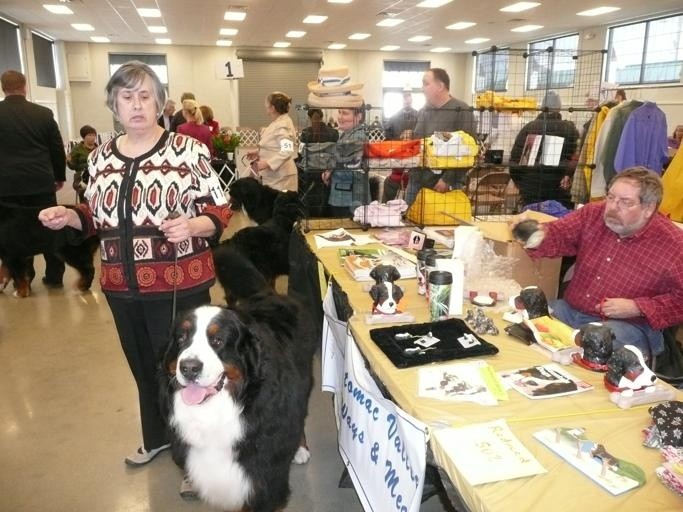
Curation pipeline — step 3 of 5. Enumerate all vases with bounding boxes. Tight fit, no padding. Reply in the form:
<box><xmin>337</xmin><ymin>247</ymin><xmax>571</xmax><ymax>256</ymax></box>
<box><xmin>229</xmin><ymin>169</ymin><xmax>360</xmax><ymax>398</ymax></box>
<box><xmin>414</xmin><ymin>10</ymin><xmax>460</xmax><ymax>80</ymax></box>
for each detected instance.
<box><xmin>216</xmin><ymin>148</ymin><xmax>234</xmax><ymax>159</ymax></box>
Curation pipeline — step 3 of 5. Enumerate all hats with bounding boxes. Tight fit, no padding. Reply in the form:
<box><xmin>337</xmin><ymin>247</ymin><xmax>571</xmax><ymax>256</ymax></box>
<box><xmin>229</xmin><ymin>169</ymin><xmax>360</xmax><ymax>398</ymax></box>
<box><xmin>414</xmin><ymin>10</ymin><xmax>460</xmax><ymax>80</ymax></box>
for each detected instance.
<box><xmin>541</xmin><ymin>91</ymin><xmax>561</xmax><ymax>108</ymax></box>
<box><xmin>307</xmin><ymin>63</ymin><xmax>363</xmax><ymax>108</ymax></box>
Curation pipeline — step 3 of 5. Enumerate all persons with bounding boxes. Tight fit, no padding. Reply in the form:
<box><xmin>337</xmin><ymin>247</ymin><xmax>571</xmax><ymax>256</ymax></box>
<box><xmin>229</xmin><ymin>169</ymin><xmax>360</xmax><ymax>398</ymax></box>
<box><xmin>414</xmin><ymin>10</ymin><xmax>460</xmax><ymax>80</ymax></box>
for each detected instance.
<box><xmin>247</xmin><ymin>92</ymin><xmax>298</xmax><ymax>192</ymax></box>
<box><xmin>0</xmin><ymin>70</ymin><xmax>67</xmax><ymax>289</ymax></box>
<box><xmin>299</xmin><ymin>109</ymin><xmax>339</xmax><ymax>143</ymax></box>
<box><xmin>511</xmin><ymin>165</ymin><xmax>683</xmax><ymax>359</ymax></box>
<box><xmin>37</xmin><ymin>60</ymin><xmax>234</xmax><ymax>503</ymax></box>
<box><xmin>508</xmin><ymin>91</ymin><xmax>579</xmax><ymax>210</ymax></box>
<box><xmin>405</xmin><ymin>70</ymin><xmax>478</xmax><ymax>206</ymax></box>
<box><xmin>387</xmin><ymin>94</ymin><xmax>418</xmax><ymax>140</ymax></box>
<box><xmin>668</xmin><ymin>125</ymin><xmax>683</xmax><ymax>141</ymax></box>
<box><xmin>322</xmin><ymin>94</ymin><xmax>372</xmax><ymax>218</ymax></box>
<box><xmin>67</xmin><ymin>125</ymin><xmax>99</xmax><ymax>203</ymax></box>
<box><xmin>157</xmin><ymin>92</ymin><xmax>221</xmax><ymax>159</ymax></box>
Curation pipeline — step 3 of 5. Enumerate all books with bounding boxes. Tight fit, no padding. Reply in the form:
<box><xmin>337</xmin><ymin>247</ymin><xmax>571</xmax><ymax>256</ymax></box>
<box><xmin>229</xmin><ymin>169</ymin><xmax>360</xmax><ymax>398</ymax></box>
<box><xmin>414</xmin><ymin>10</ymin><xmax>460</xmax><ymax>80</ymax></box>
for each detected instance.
<box><xmin>519</xmin><ymin>135</ymin><xmax>543</xmax><ymax>165</ymax></box>
<box><xmin>338</xmin><ymin>248</ymin><xmax>419</xmax><ymax>282</ymax></box>
<box><xmin>503</xmin><ymin>363</ymin><xmax>594</xmax><ymax>399</ymax></box>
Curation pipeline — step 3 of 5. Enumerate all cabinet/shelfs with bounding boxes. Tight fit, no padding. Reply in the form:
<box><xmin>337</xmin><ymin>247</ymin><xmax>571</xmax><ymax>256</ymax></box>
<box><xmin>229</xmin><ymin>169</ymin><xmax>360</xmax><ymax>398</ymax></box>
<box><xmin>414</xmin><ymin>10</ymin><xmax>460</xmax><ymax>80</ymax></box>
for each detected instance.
<box><xmin>296</xmin><ymin>47</ymin><xmax>607</xmax><ymax>234</ymax></box>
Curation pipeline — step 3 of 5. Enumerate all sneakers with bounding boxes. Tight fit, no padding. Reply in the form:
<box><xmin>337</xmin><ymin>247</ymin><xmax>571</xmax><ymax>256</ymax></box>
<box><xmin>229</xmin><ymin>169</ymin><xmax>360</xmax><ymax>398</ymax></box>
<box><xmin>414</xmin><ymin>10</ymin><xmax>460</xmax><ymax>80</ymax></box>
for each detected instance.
<box><xmin>125</xmin><ymin>441</ymin><xmax>171</xmax><ymax>467</ymax></box>
<box><xmin>180</xmin><ymin>475</ymin><xmax>202</xmax><ymax>500</ymax></box>
<box><xmin>0</xmin><ymin>263</ymin><xmax>94</xmax><ymax>298</ymax></box>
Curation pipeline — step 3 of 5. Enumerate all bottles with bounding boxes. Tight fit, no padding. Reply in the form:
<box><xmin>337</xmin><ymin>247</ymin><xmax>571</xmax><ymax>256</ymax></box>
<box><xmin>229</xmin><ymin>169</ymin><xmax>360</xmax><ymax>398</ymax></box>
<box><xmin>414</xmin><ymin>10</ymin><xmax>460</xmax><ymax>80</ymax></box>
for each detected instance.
<box><xmin>493</xmin><ymin>153</ymin><xmax>502</xmax><ymax>165</ymax></box>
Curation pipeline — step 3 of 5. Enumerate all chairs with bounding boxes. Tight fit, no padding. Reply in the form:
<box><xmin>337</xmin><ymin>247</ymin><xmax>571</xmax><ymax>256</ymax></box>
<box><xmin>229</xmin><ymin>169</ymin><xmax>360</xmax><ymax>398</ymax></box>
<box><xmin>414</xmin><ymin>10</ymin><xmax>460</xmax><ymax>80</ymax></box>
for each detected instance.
<box><xmin>469</xmin><ymin>172</ymin><xmax>511</xmax><ymax>217</ymax></box>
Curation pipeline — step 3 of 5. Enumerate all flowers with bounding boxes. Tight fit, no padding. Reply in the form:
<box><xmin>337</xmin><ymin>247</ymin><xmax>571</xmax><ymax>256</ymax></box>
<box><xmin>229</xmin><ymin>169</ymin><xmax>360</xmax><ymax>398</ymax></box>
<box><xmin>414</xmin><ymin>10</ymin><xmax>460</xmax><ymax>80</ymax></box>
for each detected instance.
<box><xmin>211</xmin><ymin>129</ymin><xmax>241</xmax><ymax>152</ymax></box>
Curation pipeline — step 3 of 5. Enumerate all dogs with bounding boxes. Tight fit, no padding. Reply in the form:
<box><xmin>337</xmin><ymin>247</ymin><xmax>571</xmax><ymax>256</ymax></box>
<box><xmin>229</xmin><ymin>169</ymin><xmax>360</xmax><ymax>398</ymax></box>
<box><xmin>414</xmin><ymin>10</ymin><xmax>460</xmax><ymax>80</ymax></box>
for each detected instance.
<box><xmin>370</xmin><ymin>262</ymin><xmax>402</xmax><ymax>283</ymax></box>
<box><xmin>227</xmin><ymin>175</ymin><xmax>283</xmax><ymax>226</ymax></box>
<box><xmin>574</xmin><ymin>321</ymin><xmax>616</xmax><ymax>371</ymax></box>
<box><xmin>76</xmin><ymin>170</ymin><xmax>89</xmax><ymax>191</ymax></box>
<box><xmin>514</xmin><ymin>286</ymin><xmax>552</xmax><ymax>323</ymax></box>
<box><xmin>153</xmin><ymin>245</ymin><xmax>317</xmax><ymax>511</ymax></box>
<box><xmin>0</xmin><ymin>205</ymin><xmax>100</xmax><ymax>298</ymax></box>
<box><xmin>605</xmin><ymin>344</ymin><xmax>657</xmax><ymax>390</ymax></box>
<box><xmin>367</xmin><ymin>281</ymin><xmax>405</xmax><ymax>316</ymax></box>
<box><xmin>213</xmin><ymin>189</ymin><xmax>313</xmax><ymax>308</ymax></box>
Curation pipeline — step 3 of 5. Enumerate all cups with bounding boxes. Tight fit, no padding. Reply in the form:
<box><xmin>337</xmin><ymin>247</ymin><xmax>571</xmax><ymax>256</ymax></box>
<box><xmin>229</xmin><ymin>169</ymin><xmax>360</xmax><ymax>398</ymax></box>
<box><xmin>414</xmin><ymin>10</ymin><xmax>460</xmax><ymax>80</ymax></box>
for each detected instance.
<box><xmin>416</xmin><ymin>249</ymin><xmax>452</xmax><ymax>323</ymax></box>
<box><xmin>227</xmin><ymin>152</ymin><xmax>233</xmax><ymax>160</ymax></box>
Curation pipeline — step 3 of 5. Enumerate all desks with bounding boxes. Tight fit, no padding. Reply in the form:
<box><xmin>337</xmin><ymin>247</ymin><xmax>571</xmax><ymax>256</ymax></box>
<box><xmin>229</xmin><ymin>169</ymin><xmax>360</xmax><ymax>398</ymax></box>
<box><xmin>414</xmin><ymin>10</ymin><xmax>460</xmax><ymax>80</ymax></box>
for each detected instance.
<box><xmin>292</xmin><ymin>211</ymin><xmax>683</xmax><ymax>512</ymax></box>
<box><xmin>211</xmin><ymin>159</ymin><xmax>236</xmax><ymax>191</ymax></box>
